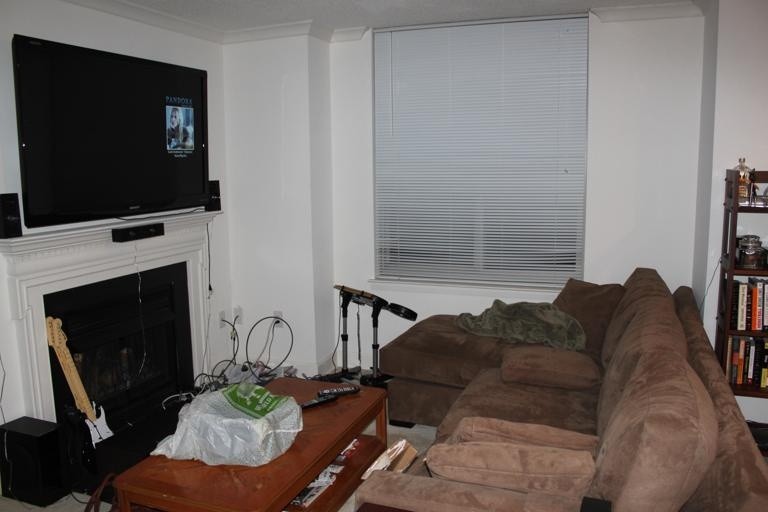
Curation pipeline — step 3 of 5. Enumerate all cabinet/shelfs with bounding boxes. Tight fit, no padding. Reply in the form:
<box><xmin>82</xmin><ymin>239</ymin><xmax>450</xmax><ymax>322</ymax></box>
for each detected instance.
<box><xmin>717</xmin><ymin>168</ymin><xmax>768</xmax><ymax>457</ymax></box>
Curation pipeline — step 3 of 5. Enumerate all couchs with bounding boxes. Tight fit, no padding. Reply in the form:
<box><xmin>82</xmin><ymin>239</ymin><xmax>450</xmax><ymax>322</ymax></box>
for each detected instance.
<box><xmin>354</xmin><ymin>268</ymin><xmax>768</xmax><ymax>512</ymax></box>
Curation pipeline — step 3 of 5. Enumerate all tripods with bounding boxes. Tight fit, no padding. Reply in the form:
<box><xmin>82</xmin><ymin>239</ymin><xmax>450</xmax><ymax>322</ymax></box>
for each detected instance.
<box><xmin>325</xmin><ymin>296</ymin><xmax>360</xmax><ymax>381</ymax></box>
<box><xmin>360</xmin><ymin>309</ymin><xmax>393</xmax><ymax>391</ymax></box>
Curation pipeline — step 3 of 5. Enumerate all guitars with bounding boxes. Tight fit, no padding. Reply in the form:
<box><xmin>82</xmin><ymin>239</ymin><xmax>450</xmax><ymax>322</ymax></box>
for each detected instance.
<box><xmin>46</xmin><ymin>317</ymin><xmax>115</xmax><ymax>471</ymax></box>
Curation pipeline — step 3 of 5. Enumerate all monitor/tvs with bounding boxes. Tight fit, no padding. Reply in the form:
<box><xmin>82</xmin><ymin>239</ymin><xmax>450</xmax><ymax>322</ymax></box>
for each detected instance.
<box><xmin>11</xmin><ymin>34</ymin><xmax>211</xmax><ymax>230</ymax></box>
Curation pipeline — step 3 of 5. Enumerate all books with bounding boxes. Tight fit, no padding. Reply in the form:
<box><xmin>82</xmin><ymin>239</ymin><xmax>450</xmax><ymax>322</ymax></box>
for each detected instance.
<box><xmin>288</xmin><ymin>463</ymin><xmax>345</xmax><ymax>508</ymax></box>
<box><xmin>730</xmin><ymin>337</ymin><xmax>768</xmax><ymax>387</ymax></box>
<box><xmin>731</xmin><ymin>277</ymin><xmax>768</xmax><ymax>331</ymax></box>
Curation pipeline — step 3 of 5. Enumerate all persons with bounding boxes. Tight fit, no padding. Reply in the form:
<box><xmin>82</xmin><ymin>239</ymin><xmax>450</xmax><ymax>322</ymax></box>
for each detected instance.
<box><xmin>165</xmin><ymin>106</ymin><xmax>190</xmax><ymax>149</ymax></box>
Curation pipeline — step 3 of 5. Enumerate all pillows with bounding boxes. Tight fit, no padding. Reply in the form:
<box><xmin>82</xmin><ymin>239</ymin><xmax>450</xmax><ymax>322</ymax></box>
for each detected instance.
<box><xmin>595</xmin><ymin>350</ymin><xmax>718</xmax><ymax>512</ymax></box>
<box><xmin>502</xmin><ymin>342</ymin><xmax>606</xmax><ymax>391</ymax></box>
<box><xmin>446</xmin><ymin>417</ymin><xmax>601</xmax><ymax>460</ymax></box>
<box><xmin>590</xmin><ymin>303</ymin><xmax>687</xmax><ymax>439</ymax></box>
<box><xmin>426</xmin><ymin>443</ymin><xmax>595</xmax><ymax>500</ymax></box>
<box><xmin>600</xmin><ymin>268</ymin><xmax>677</xmax><ymax>373</ymax></box>
<box><xmin>547</xmin><ymin>276</ymin><xmax>625</xmax><ymax>352</ymax></box>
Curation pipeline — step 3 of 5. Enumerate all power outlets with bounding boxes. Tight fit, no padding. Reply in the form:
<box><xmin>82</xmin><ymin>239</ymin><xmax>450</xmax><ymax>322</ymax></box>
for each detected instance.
<box><xmin>272</xmin><ymin>310</ymin><xmax>282</xmax><ymax>328</ymax></box>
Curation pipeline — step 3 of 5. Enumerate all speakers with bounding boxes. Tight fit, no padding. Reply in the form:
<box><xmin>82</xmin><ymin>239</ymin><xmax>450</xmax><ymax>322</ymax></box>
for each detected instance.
<box><xmin>0</xmin><ymin>415</ymin><xmax>73</xmax><ymax>508</ymax></box>
<box><xmin>203</xmin><ymin>179</ymin><xmax>222</xmax><ymax>212</ymax></box>
<box><xmin>0</xmin><ymin>191</ymin><xmax>23</xmax><ymax>239</ymax></box>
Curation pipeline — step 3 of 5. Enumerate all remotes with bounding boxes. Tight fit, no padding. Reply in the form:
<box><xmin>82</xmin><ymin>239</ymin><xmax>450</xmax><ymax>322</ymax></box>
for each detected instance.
<box><xmin>318</xmin><ymin>385</ymin><xmax>360</xmax><ymax>396</ymax></box>
<box><xmin>299</xmin><ymin>393</ymin><xmax>336</xmax><ymax>409</ymax></box>
